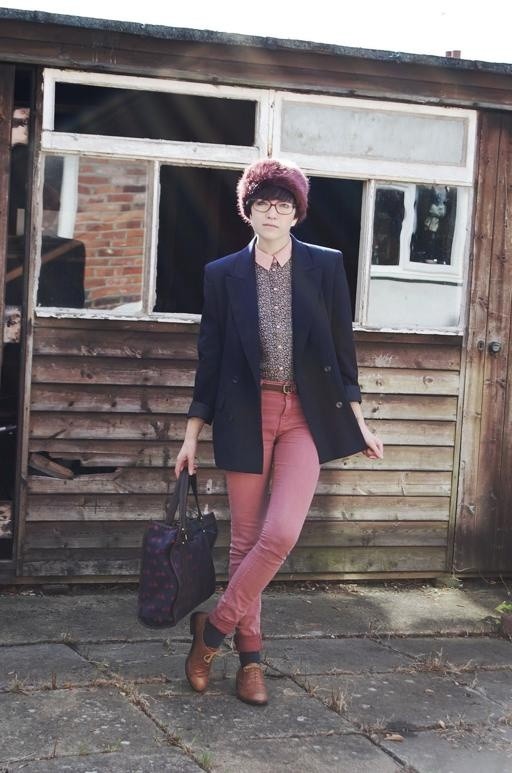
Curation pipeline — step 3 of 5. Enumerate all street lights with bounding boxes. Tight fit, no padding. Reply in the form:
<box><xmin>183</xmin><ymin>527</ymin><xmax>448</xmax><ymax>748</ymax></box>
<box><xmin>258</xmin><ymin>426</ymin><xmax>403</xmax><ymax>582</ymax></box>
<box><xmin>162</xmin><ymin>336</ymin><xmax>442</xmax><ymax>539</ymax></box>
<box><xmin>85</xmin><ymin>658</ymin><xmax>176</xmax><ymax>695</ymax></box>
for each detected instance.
<box><xmin>134</xmin><ymin>466</ymin><xmax>218</xmax><ymax>631</ymax></box>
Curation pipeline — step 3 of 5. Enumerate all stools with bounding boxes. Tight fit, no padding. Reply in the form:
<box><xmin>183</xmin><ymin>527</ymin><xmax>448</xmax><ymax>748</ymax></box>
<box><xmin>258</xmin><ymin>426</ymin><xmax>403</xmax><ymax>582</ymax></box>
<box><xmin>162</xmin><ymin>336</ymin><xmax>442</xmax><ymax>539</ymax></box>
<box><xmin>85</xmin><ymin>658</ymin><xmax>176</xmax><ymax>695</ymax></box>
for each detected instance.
<box><xmin>262</xmin><ymin>383</ymin><xmax>298</xmax><ymax>394</ymax></box>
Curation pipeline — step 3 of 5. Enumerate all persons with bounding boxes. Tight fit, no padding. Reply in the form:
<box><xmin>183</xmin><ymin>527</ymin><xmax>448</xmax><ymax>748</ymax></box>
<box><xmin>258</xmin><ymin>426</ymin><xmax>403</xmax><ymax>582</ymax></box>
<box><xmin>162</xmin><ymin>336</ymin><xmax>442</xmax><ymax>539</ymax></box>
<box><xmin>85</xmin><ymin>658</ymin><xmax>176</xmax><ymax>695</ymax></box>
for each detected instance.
<box><xmin>172</xmin><ymin>160</ymin><xmax>384</xmax><ymax>705</ymax></box>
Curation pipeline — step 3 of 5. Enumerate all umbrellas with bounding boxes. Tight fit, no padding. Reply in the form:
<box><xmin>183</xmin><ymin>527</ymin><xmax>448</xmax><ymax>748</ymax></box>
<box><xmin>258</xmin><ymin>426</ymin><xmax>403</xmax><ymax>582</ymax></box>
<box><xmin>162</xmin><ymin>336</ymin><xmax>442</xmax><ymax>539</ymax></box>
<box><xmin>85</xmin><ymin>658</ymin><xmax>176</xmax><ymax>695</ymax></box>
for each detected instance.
<box><xmin>233</xmin><ymin>663</ymin><xmax>268</xmax><ymax>708</ymax></box>
<box><xmin>185</xmin><ymin>609</ymin><xmax>220</xmax><ymax>695</ymax></box>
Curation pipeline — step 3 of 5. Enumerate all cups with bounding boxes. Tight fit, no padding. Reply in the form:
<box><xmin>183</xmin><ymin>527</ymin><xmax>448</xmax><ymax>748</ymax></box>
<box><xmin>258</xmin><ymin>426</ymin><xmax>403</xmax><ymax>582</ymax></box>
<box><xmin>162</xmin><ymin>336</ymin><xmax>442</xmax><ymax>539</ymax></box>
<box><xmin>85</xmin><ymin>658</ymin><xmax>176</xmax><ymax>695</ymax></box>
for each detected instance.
<box><xmin>250</xmin><ymin>196</ymin><xmax>297</xmax><ymax>215</ymax></box>
